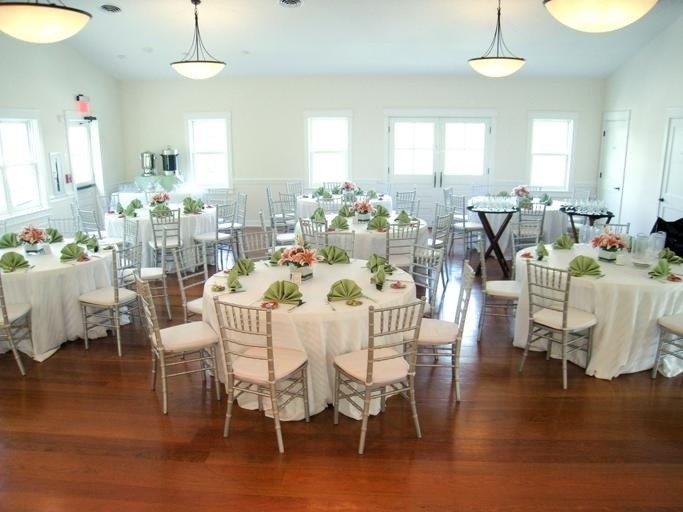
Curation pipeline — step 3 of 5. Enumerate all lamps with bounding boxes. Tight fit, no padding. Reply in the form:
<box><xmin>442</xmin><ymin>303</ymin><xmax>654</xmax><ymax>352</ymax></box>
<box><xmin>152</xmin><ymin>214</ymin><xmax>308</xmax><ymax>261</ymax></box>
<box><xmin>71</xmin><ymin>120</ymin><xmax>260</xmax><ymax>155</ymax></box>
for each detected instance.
<box><xmin>0</xmin><ymin>0</ymin><xmax>93</xmax><ymax>43</ymax></box>
<box><xmin>78</xmin><ymin>94</ymin><xmax>89</xmax><ymax>113</ymax></box>
<box><xmin>543</xmin><ymin>0</ymin><xmax>659</xmax><ymax>33</ymax></box>
<box><xmin>170</xmin><ymin>0</ymin><xmax>227</xmax><ymax>80</ymax></box>
<box><xmin>468</xmin><ymin>0</ymin><xmax>526</xmax><ymax>78</ymax></box>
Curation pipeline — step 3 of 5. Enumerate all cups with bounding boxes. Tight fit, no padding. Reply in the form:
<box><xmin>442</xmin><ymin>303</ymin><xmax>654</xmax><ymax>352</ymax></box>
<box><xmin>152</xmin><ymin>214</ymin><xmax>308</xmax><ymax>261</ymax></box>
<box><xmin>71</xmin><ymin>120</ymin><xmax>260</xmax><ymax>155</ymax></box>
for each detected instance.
<box><xmin>561</xmin><ymin>199</ymin><xmax>608</xmax><ymax>217</ymax></box>
<box><xmin>472</xmin><ymin>197</ymin><xmax>519</xmax><ymax>211</ymax></box>
